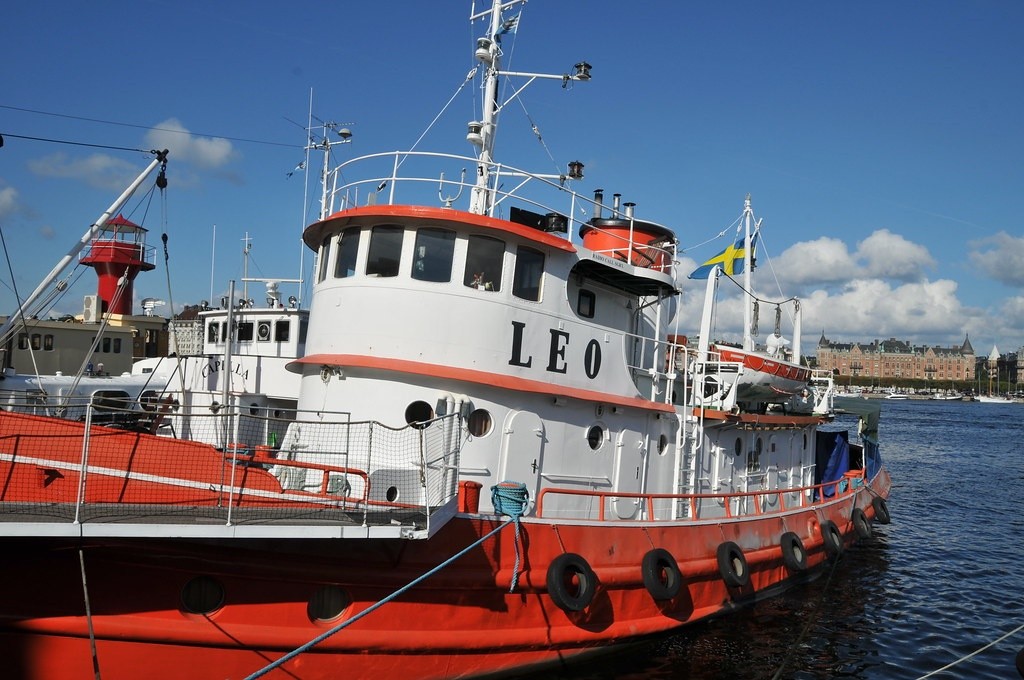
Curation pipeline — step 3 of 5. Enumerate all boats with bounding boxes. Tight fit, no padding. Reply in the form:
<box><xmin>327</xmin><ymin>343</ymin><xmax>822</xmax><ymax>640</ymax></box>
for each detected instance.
<box><xmin>0</xmin><ymin>0</ymin><xmax>1024</xmax><ymax>679</ymax></box>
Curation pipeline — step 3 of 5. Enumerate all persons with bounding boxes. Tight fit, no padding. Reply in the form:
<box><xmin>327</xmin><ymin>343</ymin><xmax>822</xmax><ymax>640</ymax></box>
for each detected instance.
<box><xmin>86</xmin><ymin>362</ymin><xmax>94</xmax><ymax>376</ymax></box>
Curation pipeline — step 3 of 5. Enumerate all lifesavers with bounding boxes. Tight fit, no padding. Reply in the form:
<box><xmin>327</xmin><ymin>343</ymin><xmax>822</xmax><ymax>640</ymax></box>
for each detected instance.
<box><xmin>716</xmin><ymin>541</ymin><xmax>750</xmax><ymax>587</ymax></box>
<box><xmin>820</xmin><ymin>520</ymin><xmax>844</xmax><ymax>557</ymax></box>
<box><xmin>872</xmin><ymin>496</ymin><xmax>890</xmax><ymax>525</ymax></box>
<box><xmin>780</xmin><ymin>531</ymin><xmax>808</xmax><ymax>571</ymax></box>
<box><xmin>547</xmin><ymin>553</ymin><xmax>598</xmax><ymax>614</ymax></box>
<box><xmin>852</xmin><ymin>508</ymin><xmax>872</xmax><ymax>539</ymax></box>
<box><xmin>641</xmin><ymin>548</ymin><xmax>682</xmax><ymax>603</ymax></box>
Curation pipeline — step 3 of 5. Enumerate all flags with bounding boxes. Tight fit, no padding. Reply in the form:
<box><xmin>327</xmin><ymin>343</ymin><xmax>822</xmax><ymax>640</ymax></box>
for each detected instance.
<box><xmin>687</xmin><ymin>232</ymin><xmax>757</xmax><ymax>279</ymax></box>
